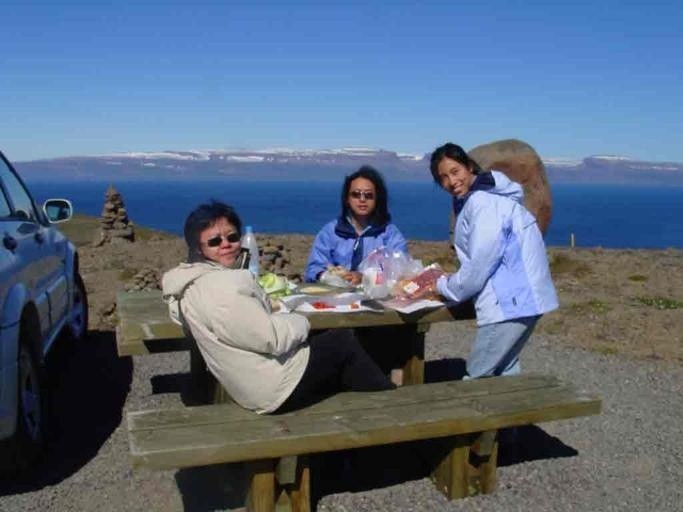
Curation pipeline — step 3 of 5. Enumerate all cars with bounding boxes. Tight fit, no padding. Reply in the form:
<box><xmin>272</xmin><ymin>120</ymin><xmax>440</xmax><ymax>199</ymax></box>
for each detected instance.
<box><xmin>0</xmin><ymin>151</ymin><xmax>88</xmax><ymax>444</ymax></box>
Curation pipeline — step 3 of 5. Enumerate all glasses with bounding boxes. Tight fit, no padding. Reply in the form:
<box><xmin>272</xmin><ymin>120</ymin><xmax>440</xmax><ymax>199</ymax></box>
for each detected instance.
<box><xmin>208</xmin><ymin>233</ymin><xmax>240</xmax><ymax>246</ymax></box>
<box><xmin>350</xmin><ymin>191</ymin><xmax>373</xmax><ymax>199</ymax></box>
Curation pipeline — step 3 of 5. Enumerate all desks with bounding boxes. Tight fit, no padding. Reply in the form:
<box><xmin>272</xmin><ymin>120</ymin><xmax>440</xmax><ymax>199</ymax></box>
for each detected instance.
<box><xmin>115</xmin><ymin>291</ymin><xmax>477</xmax><ymax>405</ymax></box>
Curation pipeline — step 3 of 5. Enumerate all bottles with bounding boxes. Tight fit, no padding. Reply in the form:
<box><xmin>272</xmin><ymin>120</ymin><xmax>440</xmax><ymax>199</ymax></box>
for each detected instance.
<box><xmin>240</xmin><ymin>225</ymin><xmax>260</xmax><ymax>281</ymax></box>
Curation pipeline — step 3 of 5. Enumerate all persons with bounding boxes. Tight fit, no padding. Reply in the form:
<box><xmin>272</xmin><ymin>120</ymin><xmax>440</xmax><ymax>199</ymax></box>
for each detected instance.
<box><xmin>304</xmin><ymin>165</ymin><xmax>410</xmax><ymax>387</ymax></box>
<box><xmin>161</xmin><ymin>199</ymin><xmax>414</xmax><ymax>488</ymax></box>
<box><xmin>429</xmin><ymin>143</ymin><xmax>560</xmax><ymax>443</ymax></box>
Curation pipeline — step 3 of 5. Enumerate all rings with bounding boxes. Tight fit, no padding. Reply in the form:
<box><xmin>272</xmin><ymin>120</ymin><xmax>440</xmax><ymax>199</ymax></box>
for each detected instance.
<box><xmin>429</xmin><ymin>288</ymin><xmax>433</xmax><ymax>292</ymax></box>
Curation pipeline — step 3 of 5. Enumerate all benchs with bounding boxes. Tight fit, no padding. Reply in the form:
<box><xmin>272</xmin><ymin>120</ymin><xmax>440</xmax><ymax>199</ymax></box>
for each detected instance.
<box><xmin>116</xmin><ymin>327</ymin><xmax>207</xmax><ymax>404</ymax></box>
<box><xmin>126</xmin><ymin>372</ymin><xmax>602</xmax><ymax>512</ymax></box>
